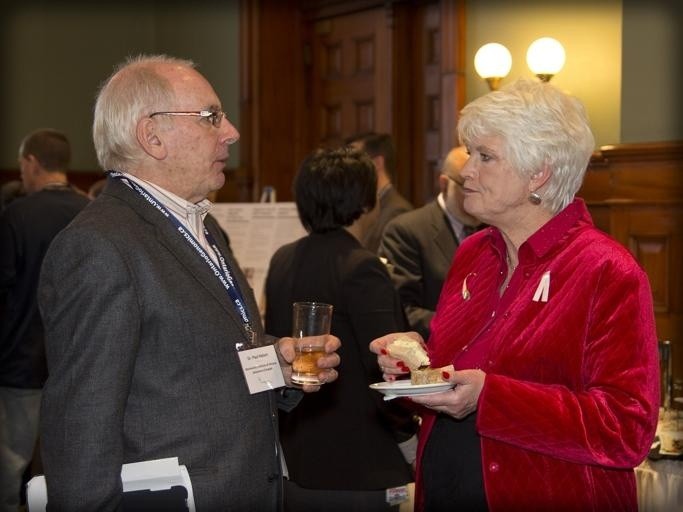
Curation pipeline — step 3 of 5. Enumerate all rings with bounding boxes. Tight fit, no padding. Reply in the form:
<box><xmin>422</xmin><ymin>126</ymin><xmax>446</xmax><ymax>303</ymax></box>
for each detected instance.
<box><xmin>379</xmin><ymin>365</ymin><xmax>384</xmax><ymax>371</ymax></box>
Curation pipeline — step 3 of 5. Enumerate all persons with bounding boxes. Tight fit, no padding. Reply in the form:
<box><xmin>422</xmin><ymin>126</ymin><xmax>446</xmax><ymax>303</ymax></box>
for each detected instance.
<box><xmin>0</xmin><ymin>129</ymin><xmax>96</xmax><ymax>511</ymax></box>
<box><xmin>366</xmin><ymin>77</ymin><xmax>663</xmax><ymax>511</ymax></box>
<box><xmin>344</xmin><ymin>132</ymin><xmax>419</xmax><ymax>260</ymax></box>
<box><xmin>84</xmin><ymin>177</ymin><xmax>106</xmax><ymax>200</ymax></box>
<box><xmin>378</xmin><ymin>145</ymin><xmax>492</xmax><ymax>346</ymax></box>
<box><xmin>261</xmin><ymin>144</ymin><xmax>419</xmax><ymax>512</ymax></box>
<box><xmin>0</xmin><ymin>178</ymin><xmax>26</xmax><ymax>208</ymax></box>
<box><xmin>38</xmin><ymin>54</ymin><xmax>344</xmax><ymax>510</ymax></box>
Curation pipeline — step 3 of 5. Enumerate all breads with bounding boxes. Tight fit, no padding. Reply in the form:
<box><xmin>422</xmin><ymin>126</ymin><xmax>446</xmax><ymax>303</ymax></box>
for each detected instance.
<box><xmin>387</xmin><ymin>337</ymin><xmax>455</xmax><ymax>385</ymax></box>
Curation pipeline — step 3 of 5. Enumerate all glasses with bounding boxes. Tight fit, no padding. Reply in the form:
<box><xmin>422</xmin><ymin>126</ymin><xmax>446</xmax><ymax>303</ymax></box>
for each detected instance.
<box><xmin>149</xmin><ymin>109</ymin><xmax>223</xmax><ymax>129</ymax></box>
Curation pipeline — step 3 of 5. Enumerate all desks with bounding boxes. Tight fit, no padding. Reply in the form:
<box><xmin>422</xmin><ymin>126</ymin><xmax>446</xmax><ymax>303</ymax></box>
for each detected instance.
<box><xmin>633</xmin><ymin>430</ymin><xmax>683</xmax><ymax>512</ymax></box>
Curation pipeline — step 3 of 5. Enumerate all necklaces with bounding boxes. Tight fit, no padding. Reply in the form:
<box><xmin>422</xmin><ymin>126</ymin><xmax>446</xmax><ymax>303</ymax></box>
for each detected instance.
<box><xmin>502</xmin><ymin>247</ymin><xmax>518</xmax><ymax>273</ymax></box>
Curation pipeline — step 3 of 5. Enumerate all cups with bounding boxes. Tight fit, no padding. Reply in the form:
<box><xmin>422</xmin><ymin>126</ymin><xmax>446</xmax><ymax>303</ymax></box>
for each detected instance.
<box><xmin>288</xmin><ymin>300</ymin><xmax>331</xmax><ymax>386</ymax></box>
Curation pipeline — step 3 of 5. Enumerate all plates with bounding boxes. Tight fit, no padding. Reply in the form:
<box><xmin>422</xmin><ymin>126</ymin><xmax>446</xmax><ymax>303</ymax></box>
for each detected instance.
<box><xmin>368</xmin><ymin>379</ymin><xmax>456</xmax><ymax>396</ymax></box>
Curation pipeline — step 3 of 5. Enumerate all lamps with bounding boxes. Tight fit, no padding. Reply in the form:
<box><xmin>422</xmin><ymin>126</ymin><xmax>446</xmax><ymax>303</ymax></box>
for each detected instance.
<box><xmin>526</xmin><ymin>39</ymin><xmax>567</xmax><ymax>83</ymax></box>
<box><xmin>474</xmin><ymin>43</ymin><xmax>512</xmax><ymax>92</ymax></box>
